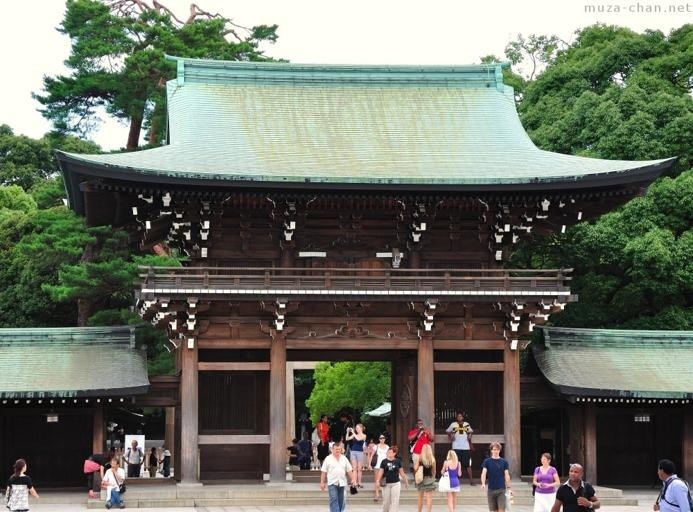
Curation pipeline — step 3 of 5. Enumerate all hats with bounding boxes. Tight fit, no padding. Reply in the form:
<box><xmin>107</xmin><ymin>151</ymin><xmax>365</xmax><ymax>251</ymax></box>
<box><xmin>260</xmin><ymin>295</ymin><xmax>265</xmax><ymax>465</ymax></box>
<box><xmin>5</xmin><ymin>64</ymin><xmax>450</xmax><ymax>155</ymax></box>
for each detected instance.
<box><xmin>163</xmin><ymin>450</ymin><xmax>171</xmax><ymax>457</ymax></box>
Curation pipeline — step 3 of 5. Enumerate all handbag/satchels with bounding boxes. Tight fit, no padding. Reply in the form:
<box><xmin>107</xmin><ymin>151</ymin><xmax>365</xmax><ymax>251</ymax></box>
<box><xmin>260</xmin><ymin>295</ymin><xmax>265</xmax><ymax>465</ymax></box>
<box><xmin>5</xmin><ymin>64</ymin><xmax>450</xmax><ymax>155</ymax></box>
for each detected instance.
<box><xmin>415</xmin><ymin>466</ymin><xmax>424</xmax><ymax>486</ymax></box>
<box><xmin>370</xmin><ymin>454</ymin><xmax>377</xmax><ymax>467</ymax></box>
<box><xmin>119</xmin><ymin>484</ymin><xmax>126</xmax><ymax>492</ymax></box>
<box><xmin>438</xmin><ymin>472</ymin><xmax>450</xmax><ymax>492</ymax></box>
<box><xmin>381</xmin><ymin>476</ymin><xmax>386</xmax><ymax>487</ymax></box>
<box><xmin>408</xmin><ymin>442</ymin><xmax>416</xmax><ymax>453</ymax></box>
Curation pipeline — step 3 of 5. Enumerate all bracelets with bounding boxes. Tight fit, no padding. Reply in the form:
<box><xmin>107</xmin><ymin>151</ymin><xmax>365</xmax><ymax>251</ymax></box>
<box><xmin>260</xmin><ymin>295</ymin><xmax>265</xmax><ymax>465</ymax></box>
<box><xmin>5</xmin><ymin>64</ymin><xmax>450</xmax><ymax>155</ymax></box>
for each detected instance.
<box><xmin>589</xmin><ymin>502</ymin><xmax>592</xmax><ymax>508</ymax></box>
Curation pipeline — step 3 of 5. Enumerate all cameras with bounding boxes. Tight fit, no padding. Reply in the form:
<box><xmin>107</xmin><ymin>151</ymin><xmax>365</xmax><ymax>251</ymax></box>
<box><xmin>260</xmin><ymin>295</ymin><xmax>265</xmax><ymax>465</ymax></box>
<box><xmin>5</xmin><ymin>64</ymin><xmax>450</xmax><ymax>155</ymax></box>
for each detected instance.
<box><xmin>348</xmin><ymin>428</ymin><xmax>352</xmax><ymax>431</ymax></box>
<box><xmin>420</xmin><ymin>427</ymin><xmax>424</xmax><ymax>430</ymax></box>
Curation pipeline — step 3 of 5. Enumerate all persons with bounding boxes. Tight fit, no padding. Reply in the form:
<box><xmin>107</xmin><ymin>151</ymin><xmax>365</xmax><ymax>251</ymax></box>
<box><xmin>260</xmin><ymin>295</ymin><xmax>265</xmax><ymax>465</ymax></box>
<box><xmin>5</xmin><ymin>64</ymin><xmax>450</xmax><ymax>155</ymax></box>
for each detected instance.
<box><xmin>159</xmin><ymin>444</ymin><xmax>171</xmax><ymax>477</ymax></box>
<box><xmin>287</xmin><ymin>413</ymin><xmax>353</xmax><ymax>470</ymax></box>
<box><xmin>441</xmin><ymin>450</ymin><xmax>462</xmax><ymax>512</ymax></box>
<box><xmin>414</xmin><ymin>444</ymin><xmax>436</xmax><ymax>512</ymax></box>
<box><xmin>445</xmin><ymin>411</ymin><xmax>476</xmax><ymax>486</ymax></box>
<box><xmin>533</xmin><ymin>453</ymin><xmax>561</xmax><ymax>512</ymax></box>
<box><xmin>368</xmin><ymin>433</ymin><xmax>390</xmax><ymax>502</ymax></box>
<box><xmin>320</xmin><ymin>443</ymin><xmax>356</xmax><ymax>512</ymax></box>
<box><xmin>480</xmin><ymin>442</ymin><xmax>510</xmax><ymax>512</ymax></box>
<box><xmin>345</xmin><ymin>423</ymin><xmax>367</xmax><ymax>488</ymax></box>
<box><xmin>149</xmin><ymin>447</ymin><xmax>158</xmax><ymax>477</ymax></box>
<box><xmin>654</xmin><ymin>459</ymin><xmax>693</xmax><ymax>511</ymax></box>
<box><xmin>122</xmin><ymin>441</ymin><xmax>145</xmax><ymax>477</ymax></box>
<box><xmin>5</xmin><ymin>459</ymin><xmax>40</xmax><ymax>512</ymax></box>
<box><xmin>376</xmin><ymin>448</ymin><xmax>409</xmax><ymax>512</ymax></box>
<box><xmin>101</xmin><ymin>457</ymin><xmax>126</xmax><ymax>509</ymax></box>
<box><xmin>408</xmin><ymin>419</ymin><xmax>434</xmax><ymax>470</ymax></box>
<box><xmin>550</xmin><ymin>463</ymin><xmax>600</xmax><ymax>512</ymax></box>
